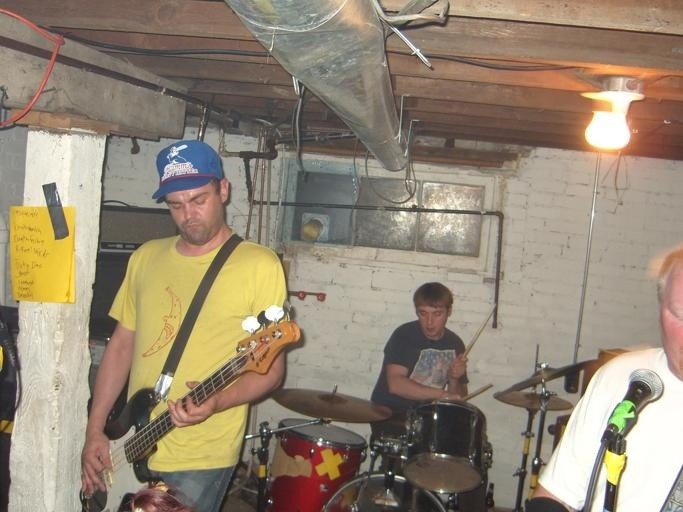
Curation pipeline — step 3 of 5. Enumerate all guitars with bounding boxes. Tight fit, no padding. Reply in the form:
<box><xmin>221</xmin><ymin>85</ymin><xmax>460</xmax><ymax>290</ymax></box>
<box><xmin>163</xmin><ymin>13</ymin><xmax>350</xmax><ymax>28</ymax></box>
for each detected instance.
<box><xmin>78</xmin><ymin>300</ymin><xmax>301</xmax><ymax>510</ymax></box>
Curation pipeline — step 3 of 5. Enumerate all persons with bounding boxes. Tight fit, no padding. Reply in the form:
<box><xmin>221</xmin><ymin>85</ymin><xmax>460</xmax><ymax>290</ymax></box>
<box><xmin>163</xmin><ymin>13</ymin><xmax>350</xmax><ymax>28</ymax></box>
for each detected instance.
<box><xmin>367</xmin><ymin>282</ymin><xmax>470</xmax><ymax>458</ymax></box>
<box><xmin>115</xmin><ymin>485</ymin><xmax>195</xmax><ymax>512</ymax></box>
<box><xmin>76</xmin><ymin>140</ymin><xmax>288</xmax><ymax>512</ymax></box>
<box><xmin>523</xmin><ymin>240</ymin><xmax>683</xmax><ymax>511</ymax></box>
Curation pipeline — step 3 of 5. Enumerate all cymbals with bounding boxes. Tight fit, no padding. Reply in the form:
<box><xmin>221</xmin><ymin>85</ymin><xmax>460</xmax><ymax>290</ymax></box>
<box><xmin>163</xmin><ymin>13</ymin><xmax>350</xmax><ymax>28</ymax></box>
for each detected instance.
<box><xmin>271</xmin><ymin>387</ymin><xmax>392</xmax><ymax>424</ymax></box>
<box><xmin>493</xmin><ymin>391</ymin><xmax>572</xmax><ymax>410</ymax></box>
<box><xmin>502</xmin><ymin>361</ymin><xmax>596</xmax><ymax>392</ymax></box>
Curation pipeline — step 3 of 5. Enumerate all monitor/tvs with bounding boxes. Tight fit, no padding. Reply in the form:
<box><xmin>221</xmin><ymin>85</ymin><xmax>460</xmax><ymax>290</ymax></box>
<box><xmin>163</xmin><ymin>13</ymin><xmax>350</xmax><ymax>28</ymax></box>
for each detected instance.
<box><xmin>89</xmin><ymin>252</ymin><xmax>135</xmax><ymax>341</ymax></box>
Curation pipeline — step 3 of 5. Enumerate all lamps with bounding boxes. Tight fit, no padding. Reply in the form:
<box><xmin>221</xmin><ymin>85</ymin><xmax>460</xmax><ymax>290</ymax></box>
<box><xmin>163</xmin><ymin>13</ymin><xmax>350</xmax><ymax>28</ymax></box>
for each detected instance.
<box><xmin>579</xmin><ymin>76</ymin><xmax>643</xmax><ymax>155</ymax></box>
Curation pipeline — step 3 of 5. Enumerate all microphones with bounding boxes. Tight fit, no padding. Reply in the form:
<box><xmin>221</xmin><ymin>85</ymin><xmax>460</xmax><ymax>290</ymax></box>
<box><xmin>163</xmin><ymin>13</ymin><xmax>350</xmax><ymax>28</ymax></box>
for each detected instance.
<box><xmin>0</xmin><ymin>308</ymin><xmax>24</xmax><ymax>371</ymax></box>
<box><xmin>601</xmin><ymin>368</ymin><xmax>663</xmax><ymax>447</ymax></box>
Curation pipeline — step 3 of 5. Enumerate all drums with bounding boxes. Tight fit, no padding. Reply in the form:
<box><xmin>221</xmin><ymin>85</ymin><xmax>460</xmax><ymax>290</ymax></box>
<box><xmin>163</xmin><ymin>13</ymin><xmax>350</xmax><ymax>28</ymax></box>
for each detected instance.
<box><xmin>322</xmin><ymin>475</ymin><xmax>447</xmax><ymax>512</ymax></box>
<box><xmin>402</xmin><ymin>400</ymin><xmax>486</xmax><ymax>494</ymax></box>
<box><xmin>265</xmin><ymin>418</ymin><xmax>366</xmax><ymax>512</ymax></box>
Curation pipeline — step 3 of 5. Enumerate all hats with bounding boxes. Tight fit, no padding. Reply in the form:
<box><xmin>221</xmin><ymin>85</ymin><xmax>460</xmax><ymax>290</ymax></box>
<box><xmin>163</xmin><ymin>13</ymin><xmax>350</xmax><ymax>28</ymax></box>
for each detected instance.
<box><xmin>152</xmin><ymin>139</ymin><xmax>225</xmax><ymax>200</ymax></box>
